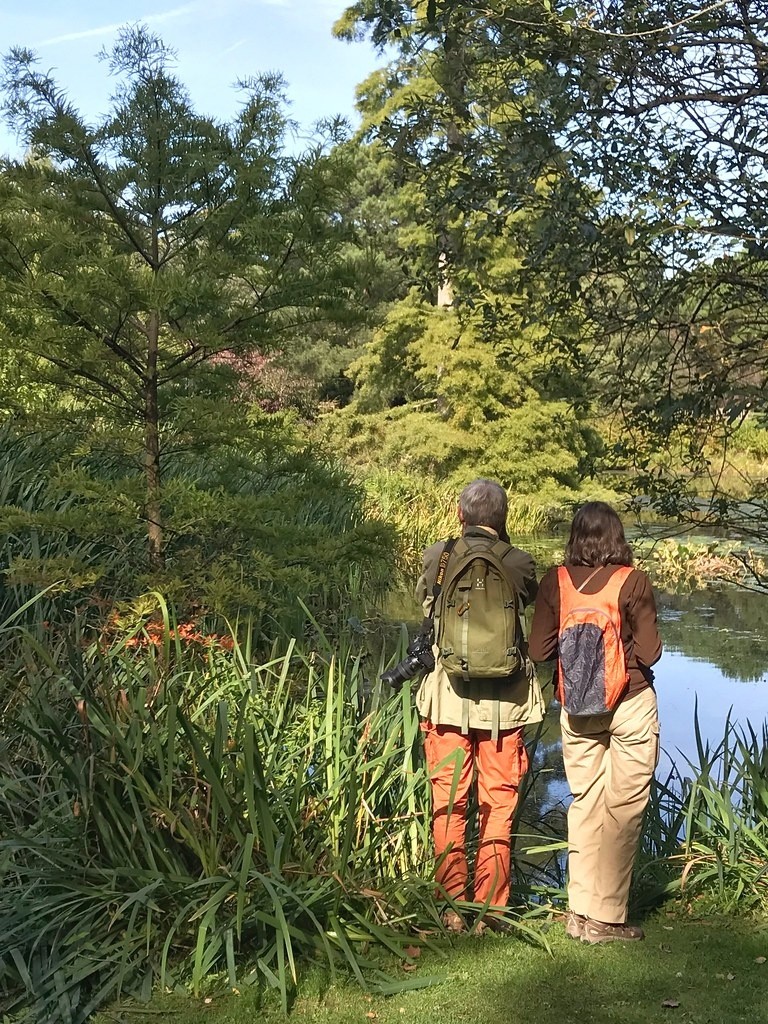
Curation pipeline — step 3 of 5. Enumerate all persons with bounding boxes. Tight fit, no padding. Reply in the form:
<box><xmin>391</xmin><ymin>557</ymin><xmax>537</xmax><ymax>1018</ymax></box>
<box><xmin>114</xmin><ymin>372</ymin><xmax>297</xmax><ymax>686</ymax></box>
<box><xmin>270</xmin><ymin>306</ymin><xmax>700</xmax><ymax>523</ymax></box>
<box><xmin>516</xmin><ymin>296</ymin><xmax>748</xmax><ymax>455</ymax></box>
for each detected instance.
<box><xmin>527</xmin><ymin>501</ymin><xmax>663</xmax><ymax>944</ymax></box>
<box><xmin>414</xmin><ymin>478</ymin><xmax>546</xmax><ymax>931</ymax></box>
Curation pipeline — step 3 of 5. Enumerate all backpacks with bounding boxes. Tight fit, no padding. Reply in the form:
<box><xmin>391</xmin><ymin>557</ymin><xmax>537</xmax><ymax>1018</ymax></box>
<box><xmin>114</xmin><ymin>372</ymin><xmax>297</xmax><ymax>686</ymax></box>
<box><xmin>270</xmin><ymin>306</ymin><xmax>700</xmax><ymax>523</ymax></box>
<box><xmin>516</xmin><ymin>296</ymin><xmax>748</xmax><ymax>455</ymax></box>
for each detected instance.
<box><xmin>553</xmin><ymin>566</ymin><xmax>635</xmax><ymax>716</ymax></box>
<box><xmin>437</xmin><ymin>537</ymin><xmax>526</xmax><ymax>679</ymax></box>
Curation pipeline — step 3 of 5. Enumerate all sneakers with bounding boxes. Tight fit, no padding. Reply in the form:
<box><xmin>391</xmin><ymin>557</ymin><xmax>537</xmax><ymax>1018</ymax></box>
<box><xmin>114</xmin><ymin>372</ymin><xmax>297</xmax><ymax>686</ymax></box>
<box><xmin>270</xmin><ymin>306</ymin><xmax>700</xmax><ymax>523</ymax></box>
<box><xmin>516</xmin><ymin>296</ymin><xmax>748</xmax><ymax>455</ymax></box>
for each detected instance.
<box><xmin>566</xmin><ymin>915</ymin><xmax>585</xmax><ymax>938</ymax></box>
<box><xmin>580</xmin><ymin>917</ymin><xmax>643</xmax><ymax>943</ymax></box>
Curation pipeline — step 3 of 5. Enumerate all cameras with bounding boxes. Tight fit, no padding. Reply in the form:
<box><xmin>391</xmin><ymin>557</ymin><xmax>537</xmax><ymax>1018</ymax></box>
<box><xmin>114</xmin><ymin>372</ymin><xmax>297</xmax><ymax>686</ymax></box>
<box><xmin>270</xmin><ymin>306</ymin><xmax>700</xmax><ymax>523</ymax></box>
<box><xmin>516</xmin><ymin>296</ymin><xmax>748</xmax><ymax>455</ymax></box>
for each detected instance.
<box><xmin>379</xmin><ymin>640</ymin><xmax>435</xmax><ymax>693</ymax></box>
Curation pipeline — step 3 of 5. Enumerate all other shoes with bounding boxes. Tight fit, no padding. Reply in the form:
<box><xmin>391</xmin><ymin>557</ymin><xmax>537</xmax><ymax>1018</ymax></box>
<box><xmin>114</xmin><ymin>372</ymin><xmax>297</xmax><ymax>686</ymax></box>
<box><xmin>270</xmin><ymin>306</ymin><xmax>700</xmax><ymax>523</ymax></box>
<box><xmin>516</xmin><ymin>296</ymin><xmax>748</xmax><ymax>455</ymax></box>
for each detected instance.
<box><xmin>443</xmin><ymin>912</ymin><xmax>467</xmax><ymax>926</ymax></box>
<box><xmin>474</xmin><ymin>912</ymin><xmax>501</xmax><ymax>926</ymax></box>
